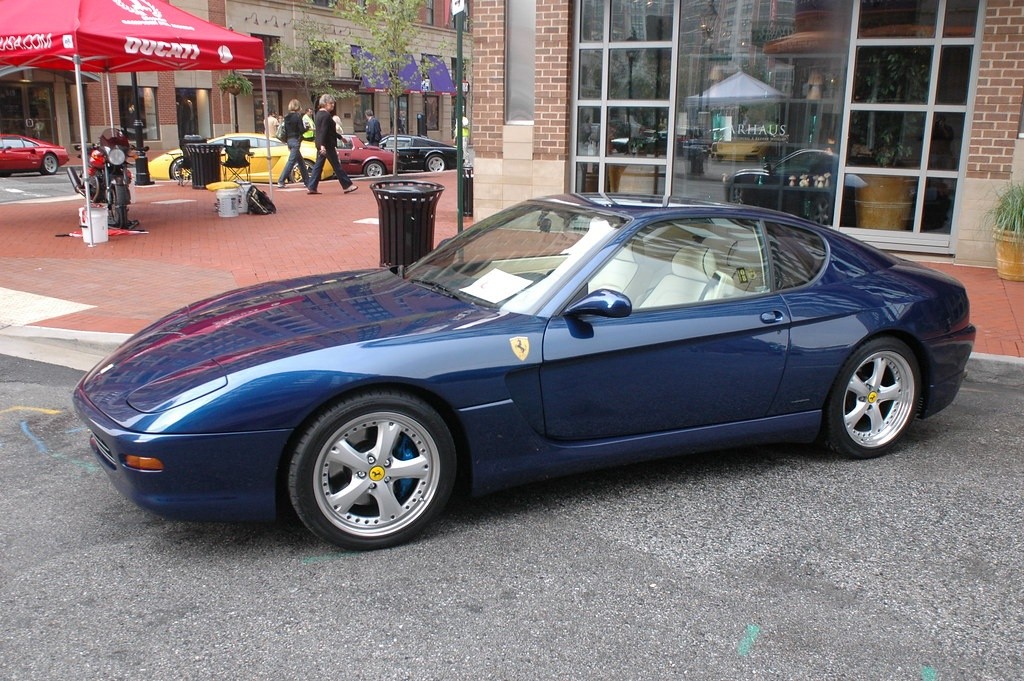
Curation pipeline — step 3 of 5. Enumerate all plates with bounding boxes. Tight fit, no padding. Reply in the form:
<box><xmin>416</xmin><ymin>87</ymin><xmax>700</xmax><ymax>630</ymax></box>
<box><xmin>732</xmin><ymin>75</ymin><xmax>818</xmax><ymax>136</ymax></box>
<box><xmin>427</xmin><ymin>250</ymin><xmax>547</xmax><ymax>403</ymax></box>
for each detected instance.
<box><xmin>84</xmin><ymin>203</ymin><xmax>108</xmax><ymax>209</ymax></box>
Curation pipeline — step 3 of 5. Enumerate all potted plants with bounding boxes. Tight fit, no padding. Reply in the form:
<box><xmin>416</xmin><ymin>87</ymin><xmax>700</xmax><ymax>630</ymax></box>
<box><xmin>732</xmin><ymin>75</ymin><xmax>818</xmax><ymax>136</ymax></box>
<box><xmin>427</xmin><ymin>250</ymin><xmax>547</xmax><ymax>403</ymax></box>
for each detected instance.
<box><xmin>990</xmin><ymin>185</ymin><xmax>1024</xmax><ymax>281</ymax></box>
<box><xmin>217</xmin><ymin>73</ymin><xmax>254</xmax><ymax>97</ymax></box>
<box><xmin>854</xmin><ymin>36</ymin><xmax>946</xmax><ymax>231</ymax></box>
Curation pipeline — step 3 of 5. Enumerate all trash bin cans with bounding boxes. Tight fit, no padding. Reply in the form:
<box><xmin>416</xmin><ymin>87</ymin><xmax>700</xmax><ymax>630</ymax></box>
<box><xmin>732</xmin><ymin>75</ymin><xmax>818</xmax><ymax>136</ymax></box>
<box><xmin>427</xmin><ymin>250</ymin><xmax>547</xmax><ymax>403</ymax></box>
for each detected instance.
<box><xmin>461</xmin><ymin>167</ymin><xmax>474</xmax><ymax>217</ymax></box>
<box><xmin>216</xmin><ymin>189</ymin><xmax>239</xmax><ymax>217</ymax></box>
<box><xmin>238</xmin><ymin>185</ymin><xmax>252</xmax><ymax>213</ymax></box>
<box><xmin>79</xmin><ymin>207</ymin><xmax>109</xmax><ymax>244</ymax></box>
<box><xmin>631</xmin><ymin>135</ymin><xmax>647</xmax><ymax>155</ymax></box>
<box><xmin>370</xmin><ymin>180</ymin><xmax>445</xmax><ymax>267</ymax></box>
<box><xmin>183</xmin><ymin>142</ymin><xmax>224</xmax><ymax>190</ymax></box>
<box><xmin>689</xmin><ymin>145</ymin><xmax>709</xmax><ymax>175</ymax></box>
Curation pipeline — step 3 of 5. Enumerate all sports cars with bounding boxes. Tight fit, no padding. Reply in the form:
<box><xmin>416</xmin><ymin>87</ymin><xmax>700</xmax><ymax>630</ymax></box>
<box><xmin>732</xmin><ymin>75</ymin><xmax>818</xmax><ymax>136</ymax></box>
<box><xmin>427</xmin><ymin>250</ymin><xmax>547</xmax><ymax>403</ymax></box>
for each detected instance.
<box><xmin>0</xmin><ymin>133</ymin><xmax>70</xmax><ymax>178</ymax></box>
<box><xmin>148</xmin><ymin>133</ymin><xmax>341</xmax><ymax>183</ymax></box>
<box><xmin>72</xmin><ymin>194</ymin><xmax>978</xmax><ymax>552</ymax></box>
<box><xmin>711</xmin><ymin>135</ymin><xmax>779</xmax><ymax>163</ymax></box>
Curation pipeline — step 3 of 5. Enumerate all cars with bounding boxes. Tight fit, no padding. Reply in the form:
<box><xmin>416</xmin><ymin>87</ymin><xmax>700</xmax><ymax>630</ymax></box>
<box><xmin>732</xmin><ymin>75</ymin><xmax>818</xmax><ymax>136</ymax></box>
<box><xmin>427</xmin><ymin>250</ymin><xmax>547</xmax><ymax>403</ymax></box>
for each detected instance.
<box><xmin>724</xmin><ymin>147</ymin><xmax>950</xmax><ymax>231</ymax></box>
<box><xmin>363</xmin><ymin>134</ymin><xmax>465</xmax><ymax>172</ymax></box>
<box><xmin>611</xmin><ymin>128</ymin><xmax>723</xmax><ymax>160</ymax></box>
<box><xmin>336</xmin><ymin>134</ymin><xmax>393</xmax><ymax>177</ymax></box>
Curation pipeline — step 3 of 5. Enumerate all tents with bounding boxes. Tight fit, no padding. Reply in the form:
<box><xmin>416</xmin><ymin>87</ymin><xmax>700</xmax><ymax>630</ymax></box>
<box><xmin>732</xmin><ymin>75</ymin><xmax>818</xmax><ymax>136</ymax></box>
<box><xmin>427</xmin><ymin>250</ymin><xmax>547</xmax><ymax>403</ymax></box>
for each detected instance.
<box><xmin>0</xmin><ymin>0</ymin><xmax>274</xmax><ymax>250</ymax></box>
<box><xmin>683</xmin><ymin>71</ymin><xmax>786</xmax><ymax>160</ymax></box>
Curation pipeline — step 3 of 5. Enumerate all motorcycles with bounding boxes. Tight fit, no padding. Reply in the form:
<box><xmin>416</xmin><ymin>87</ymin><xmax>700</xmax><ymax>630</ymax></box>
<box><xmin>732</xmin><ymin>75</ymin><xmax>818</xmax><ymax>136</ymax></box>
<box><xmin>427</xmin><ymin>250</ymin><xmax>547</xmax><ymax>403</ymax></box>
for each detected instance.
<box><xmin>67</xmin><ymin>128</ymin><xmax>149</xmax><ymax>229</ymax></box>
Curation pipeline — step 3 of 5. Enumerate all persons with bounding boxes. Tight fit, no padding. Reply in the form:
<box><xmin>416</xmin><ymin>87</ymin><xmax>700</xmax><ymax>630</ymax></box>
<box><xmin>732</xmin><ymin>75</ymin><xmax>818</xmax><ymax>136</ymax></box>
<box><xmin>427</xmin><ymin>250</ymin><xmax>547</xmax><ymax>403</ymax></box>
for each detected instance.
<box><xmin>398</xmin><ymin>111</ymin><xmax>407</xmax><ymax>134</ymax></box>
<box><xmin>333</xmin><ymin>116</ymin><xmax>344</xmax><ymax>134</ymax></box>
<box><xmin>183</xmin><ymin>99</ymin><xmax>195</xmax><ymax>135</ymax></box>
<box><xmin>454</xmin><ymin>117</ymin><xmax>471</xmax><ymax>162</ymax></box>
<box><xmin>301</xmin><ymin>107</ymin><xmax>317</xmax><ymax>130</ymax></box>
<box><xmin>262</xmin><ymin>112</ymin><xmax>284</xmax><ymax>136</ymax></box>
<box><xmin>176</xmin><ymin>102</ymin><xmax>186</xmax><ymax>138</ymax></box>
<box><xmin>365</xmin><ymin>109</ymin><xmax>382</xmax><ymax>147</ymax></box>
<box><xmin>276</xmin><ymin>98</ymin><xmax>310</xmax><ymax>188</ymax></box>
<box><xmin>305</xmin><ymin>93</ymin><xmax>358</xmax><ymax>193</ymax></box>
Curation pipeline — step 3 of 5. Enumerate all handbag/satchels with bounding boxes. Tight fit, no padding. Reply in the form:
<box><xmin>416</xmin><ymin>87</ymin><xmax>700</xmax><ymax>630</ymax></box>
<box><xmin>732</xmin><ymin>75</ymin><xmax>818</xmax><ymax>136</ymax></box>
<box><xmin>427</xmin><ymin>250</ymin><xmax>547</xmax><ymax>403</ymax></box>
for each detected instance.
<box><xmin>247</xmin><ymin>184</ymin><xmax>276</xmax><ymax>214</ymax></box>
<box><xmin>275</xmin><ymin>120</ymin><xmax>287</xmax><ymax>143</ymax></box>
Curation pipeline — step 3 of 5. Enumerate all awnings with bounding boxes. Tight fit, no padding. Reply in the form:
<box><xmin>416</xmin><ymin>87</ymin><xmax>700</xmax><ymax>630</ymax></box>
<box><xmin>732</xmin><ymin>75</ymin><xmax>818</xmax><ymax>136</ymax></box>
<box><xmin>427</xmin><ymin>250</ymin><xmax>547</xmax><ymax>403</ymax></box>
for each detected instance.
<box><xmin>351</xmin><ymin>46</ymin><xmax>393</xmax><ymax>92</ymax></box>
<box><xmin>388</xmin><ymin>52</ymin><xmax>426</xmax><ymax>94</ymax></box>
<box><xmin>421</xmin><ymin>55</ymin><xmax>456</xmax><ymax>96</ymax></box>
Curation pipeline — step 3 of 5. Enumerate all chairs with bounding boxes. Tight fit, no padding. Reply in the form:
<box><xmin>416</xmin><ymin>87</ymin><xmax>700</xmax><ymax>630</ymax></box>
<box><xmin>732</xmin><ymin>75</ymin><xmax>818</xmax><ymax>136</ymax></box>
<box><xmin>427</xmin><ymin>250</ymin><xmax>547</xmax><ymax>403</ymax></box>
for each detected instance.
<box><xmin>588</xmin><ymin>233</ymin><xmax>648</xmax><ymax>295</ymax></box>
<box><xmin>640</xmin><ymin>242</ymin><xmax>735</xmax><ymax>311</ymax></box>
<box><xmin>166</xmin><ymin>138</ymin><xmax>206</xmax><ymax>186</ymax></box>
<box><xmin>223</xmin><ymin>139</ymin><xmax>254</xmax><ymax>183</ymax></box>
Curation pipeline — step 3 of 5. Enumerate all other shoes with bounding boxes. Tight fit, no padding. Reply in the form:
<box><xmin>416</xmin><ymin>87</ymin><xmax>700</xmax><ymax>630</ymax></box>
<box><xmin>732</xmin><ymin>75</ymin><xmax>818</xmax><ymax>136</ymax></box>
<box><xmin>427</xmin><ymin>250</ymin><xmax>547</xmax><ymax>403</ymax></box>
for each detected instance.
<box><xmin>278</xmin><ymin>183</ymin><xmax>285</xmax><ymax>188</ymax></box>
<box><xmin>305</xmin><ymin>185</ymin><xmax>322</xmax><ymax>194</ymax></box>
<box><xmin>344</xmin><ymin>186</ymin><xmax>358</xmax><ymax>193</ymax></box>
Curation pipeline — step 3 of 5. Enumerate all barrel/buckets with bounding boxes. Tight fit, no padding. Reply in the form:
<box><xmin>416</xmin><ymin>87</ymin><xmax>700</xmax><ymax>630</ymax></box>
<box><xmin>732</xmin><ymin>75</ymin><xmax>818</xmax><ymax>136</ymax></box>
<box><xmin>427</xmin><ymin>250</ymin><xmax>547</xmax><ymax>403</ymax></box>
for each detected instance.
<box><xmin>237</xmin><ymin>181</ymin><xmax>252</xmax><ymax>213</ymax></box>
<box><xmin>216</xmin><ymin>188</ymin><xmax>239</xmax><ymax>217</ymax></box>
<box><xmin>79</xmin><ymin>208</ymin><xmax>109</xmax><ymax>244</ymax></box>
